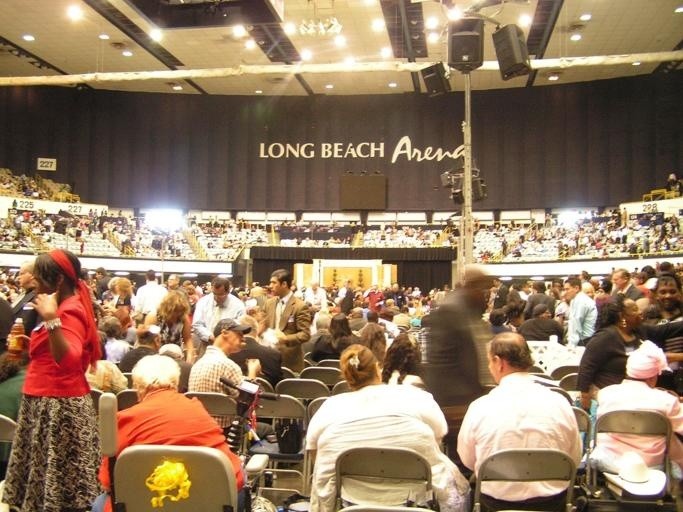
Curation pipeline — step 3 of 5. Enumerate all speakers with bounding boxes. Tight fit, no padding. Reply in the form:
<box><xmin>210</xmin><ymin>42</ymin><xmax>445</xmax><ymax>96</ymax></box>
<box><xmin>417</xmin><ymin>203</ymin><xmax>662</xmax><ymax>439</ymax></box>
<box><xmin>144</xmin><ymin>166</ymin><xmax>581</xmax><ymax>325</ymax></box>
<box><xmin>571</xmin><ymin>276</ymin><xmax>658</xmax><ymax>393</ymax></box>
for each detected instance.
<box><xmin>452</xmin><ymin>189</ymin><xmax>464</xmax><ymax>204</ymax></box>
<box><xmin>420</xmin><ymin>61</ymin><xmax>451</xmax><ymax>98</ymax></box>
<box><xmin>492</xmin><ymin>24</ymin><xmax>531</xmax><ymax>81</ymax></box>
<box><xmin>448</xmin><ymin>19</ymin><xmax>485</xmax><ymax>74</ymax></box>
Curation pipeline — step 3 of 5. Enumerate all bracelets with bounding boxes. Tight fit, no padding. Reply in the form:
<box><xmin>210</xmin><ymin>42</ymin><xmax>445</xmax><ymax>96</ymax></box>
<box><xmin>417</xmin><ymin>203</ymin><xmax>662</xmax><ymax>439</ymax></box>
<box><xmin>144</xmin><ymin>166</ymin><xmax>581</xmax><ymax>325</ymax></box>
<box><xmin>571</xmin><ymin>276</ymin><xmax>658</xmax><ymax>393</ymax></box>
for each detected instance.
<box><xmin>43</xmin><ymin>315</ymin><xmax>62</xmax><ymax>331</ymax></box>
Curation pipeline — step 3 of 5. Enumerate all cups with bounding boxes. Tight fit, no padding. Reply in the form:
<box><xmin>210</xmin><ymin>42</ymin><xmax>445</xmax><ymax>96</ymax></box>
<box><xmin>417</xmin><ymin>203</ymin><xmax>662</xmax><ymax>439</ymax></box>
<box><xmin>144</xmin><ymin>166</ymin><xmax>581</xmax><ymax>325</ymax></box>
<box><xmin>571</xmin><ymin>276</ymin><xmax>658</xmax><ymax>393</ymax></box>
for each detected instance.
<box><xmin>249</xmin><ymin>358</ymin><xmax>259</xmax><ymax>371</ymax></box>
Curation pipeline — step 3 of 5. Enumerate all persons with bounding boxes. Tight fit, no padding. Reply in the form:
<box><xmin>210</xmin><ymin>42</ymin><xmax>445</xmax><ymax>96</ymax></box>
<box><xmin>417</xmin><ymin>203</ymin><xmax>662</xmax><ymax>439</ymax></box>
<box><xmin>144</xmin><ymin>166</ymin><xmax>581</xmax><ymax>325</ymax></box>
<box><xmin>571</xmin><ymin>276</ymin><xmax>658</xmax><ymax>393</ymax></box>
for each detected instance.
<box><xmin>91</xmin><ymin>357</ymin><xmax>252</xmax><ymax>512</ymax></box>
<box><xmin>296</xmin><ymin>343</ymin><xmax>471</xmax><ymax>511</ymax></box>
<box><xmin>456</xmin><ymin>328</ymin><xmax>584</xmax><ymax>512</ymax></box>
<box><xmin>2</xmin><ymin>248</ymin><xmax>113</xmax><ymax>512</ymax></box>
<box><xmin>0</xmin><ymin>170</ymin><xmax>683</xmax><ymax>475</ymax></box>
<box><xmin>593</xmin><ymin>338</ymin><xmax>682</xmax><ymax>512</ymax></box>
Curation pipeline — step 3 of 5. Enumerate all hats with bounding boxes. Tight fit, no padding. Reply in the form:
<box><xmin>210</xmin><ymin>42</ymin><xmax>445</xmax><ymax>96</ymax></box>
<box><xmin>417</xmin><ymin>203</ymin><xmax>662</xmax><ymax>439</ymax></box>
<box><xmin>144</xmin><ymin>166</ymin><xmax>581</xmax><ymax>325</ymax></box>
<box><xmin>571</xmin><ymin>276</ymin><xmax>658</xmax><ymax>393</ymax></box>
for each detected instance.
<box><xmin>214</xmin><ymin>318</ymin><xmax>251</xmax><ymax>339</ymax></box>
<box><xmin>604</xmin><ymin>453</ymin><xmax>666</xmax><ymax>497</ymax></box>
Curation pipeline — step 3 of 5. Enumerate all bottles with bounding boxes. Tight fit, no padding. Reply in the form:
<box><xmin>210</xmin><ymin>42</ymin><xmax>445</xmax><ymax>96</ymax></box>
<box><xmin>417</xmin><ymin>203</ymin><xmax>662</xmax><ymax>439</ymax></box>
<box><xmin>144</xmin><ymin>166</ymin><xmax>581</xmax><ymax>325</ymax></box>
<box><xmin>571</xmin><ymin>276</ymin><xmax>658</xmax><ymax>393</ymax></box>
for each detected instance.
<box><xmin>5</xmin><ymin>317</ymin><xmax>25</xmax><ymax>360</ymax></box>
<box><xmin>549</xmin><ymin>334</ymin><xmax>558</xmax><ymax>355</ymax></box>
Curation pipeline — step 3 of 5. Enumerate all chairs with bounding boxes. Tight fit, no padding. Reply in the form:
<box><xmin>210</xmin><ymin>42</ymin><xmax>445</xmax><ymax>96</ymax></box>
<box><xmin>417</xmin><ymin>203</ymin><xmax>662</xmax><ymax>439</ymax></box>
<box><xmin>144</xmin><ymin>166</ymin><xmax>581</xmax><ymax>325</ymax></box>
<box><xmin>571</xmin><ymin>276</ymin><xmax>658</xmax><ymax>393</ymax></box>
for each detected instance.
<box><xmin>111</xmin><ymin>445</ymin><xmax>237</xmax><ymax>512</ymax></box>
<box><xmin>473</xmin><ymin>449</ymin><xmax>576</xmax><ymax>511</ymax></box>
<box><xmin>333</xmin><ymin>446</ymin><xmax>435</xmax><ymax>510</ymax></box>
<box><xmin>0</xmin><ymin>316</ymin><xmax>682</xmax><ymax>488</ymax></box>
<box><xmin>0</xmin><ymin>170</ymin><xmax>683</xmax><ymax>262</ymax></box>
<box><xmin>589</xmin><ymin>409</ymin><xmax>674</xmax><ymax>502</ymax></box>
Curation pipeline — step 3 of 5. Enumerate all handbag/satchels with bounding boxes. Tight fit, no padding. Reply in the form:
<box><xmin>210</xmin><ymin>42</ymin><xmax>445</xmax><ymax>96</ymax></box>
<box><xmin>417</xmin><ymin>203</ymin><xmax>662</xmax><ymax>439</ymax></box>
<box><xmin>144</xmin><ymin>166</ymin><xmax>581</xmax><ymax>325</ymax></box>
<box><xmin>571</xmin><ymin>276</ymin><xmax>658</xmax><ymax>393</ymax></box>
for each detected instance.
<box><xmin>275</xmin><ymin>423</ymin><xmax>302</xmax><ymax>453</ymax></box>
